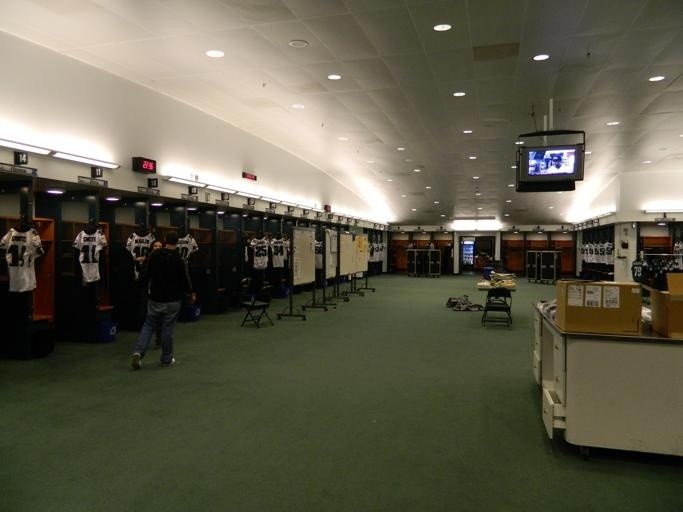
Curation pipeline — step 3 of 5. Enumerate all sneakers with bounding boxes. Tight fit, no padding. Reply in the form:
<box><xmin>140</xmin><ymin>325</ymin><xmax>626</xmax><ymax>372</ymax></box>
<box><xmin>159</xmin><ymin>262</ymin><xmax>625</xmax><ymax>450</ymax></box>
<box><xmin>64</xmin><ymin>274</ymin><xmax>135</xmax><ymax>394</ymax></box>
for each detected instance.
<box><xmin>132</xmin><ymin>353</ymin><xmax>141</xmax><ymax>370</ymax></box>
<box><xmin>160</xmin><ymin>358</ymin><xmax>176</xmax><ymax>367</ymax></box>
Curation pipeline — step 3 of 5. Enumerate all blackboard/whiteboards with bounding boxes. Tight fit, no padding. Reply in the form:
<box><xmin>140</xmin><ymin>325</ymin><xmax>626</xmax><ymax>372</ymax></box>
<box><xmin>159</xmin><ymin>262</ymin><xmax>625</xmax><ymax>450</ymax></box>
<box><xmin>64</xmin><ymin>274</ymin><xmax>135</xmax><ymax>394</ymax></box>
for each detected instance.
<box><xmin>292</xmin><ymin>227</ymin><xmax>315</xmax><ymax>286</ymax></box>
<box><xmin>322</xmin><ymin>228</ymin><xmax>368</xmax><ymax>280</ymax></box>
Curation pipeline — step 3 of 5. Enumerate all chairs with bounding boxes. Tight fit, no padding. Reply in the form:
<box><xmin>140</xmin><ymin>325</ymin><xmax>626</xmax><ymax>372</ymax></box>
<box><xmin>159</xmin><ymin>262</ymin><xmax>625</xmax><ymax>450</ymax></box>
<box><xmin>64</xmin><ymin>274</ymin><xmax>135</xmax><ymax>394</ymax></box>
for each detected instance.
<box><xmin>243</xmin><ymin>281</ymin><xmax>276</xmax><ymax>329</ymax></box>
<box><xmin>478</xmin><ymin>284</ymin><xmax>514</xmax><ymax>328</ymax></box>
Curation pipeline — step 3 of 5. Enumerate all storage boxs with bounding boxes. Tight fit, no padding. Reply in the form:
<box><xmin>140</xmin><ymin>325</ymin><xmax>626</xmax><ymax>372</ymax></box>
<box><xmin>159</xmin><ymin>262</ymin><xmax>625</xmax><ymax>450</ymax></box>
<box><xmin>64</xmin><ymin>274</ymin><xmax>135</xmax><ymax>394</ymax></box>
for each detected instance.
<box><xmin>554</xmin><ymin>269</ymin><xmax>682</xmax><ymax>337</ymax></box>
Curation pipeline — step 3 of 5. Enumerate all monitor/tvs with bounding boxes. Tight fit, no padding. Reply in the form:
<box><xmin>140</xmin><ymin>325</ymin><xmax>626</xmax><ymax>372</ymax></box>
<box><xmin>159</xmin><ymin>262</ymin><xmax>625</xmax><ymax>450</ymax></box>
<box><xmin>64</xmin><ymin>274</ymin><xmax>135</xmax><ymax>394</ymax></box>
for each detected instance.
<box><xmin>519</xmin><ymin>144</ymin><xmax>585</xmax><ymax>181</ymax></box>
<box><xmin>516</xmin><ymin>161</ymin><xmax>576</xmax><ymax>193</ymax></box>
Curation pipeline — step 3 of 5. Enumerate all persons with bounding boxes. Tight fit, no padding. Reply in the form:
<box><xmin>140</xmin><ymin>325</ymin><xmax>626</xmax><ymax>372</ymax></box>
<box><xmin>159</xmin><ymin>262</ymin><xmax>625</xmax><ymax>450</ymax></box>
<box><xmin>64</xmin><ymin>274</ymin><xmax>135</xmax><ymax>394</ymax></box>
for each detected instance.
<box><xmin>134</xmin><ymin>240</ymin><xmax>165</xmax><ymax>350</ymax></box>
<box><xmin>131</xmin><ymin>232</ymin><xmax>196</xmax><ymax>371</ymax></box>
